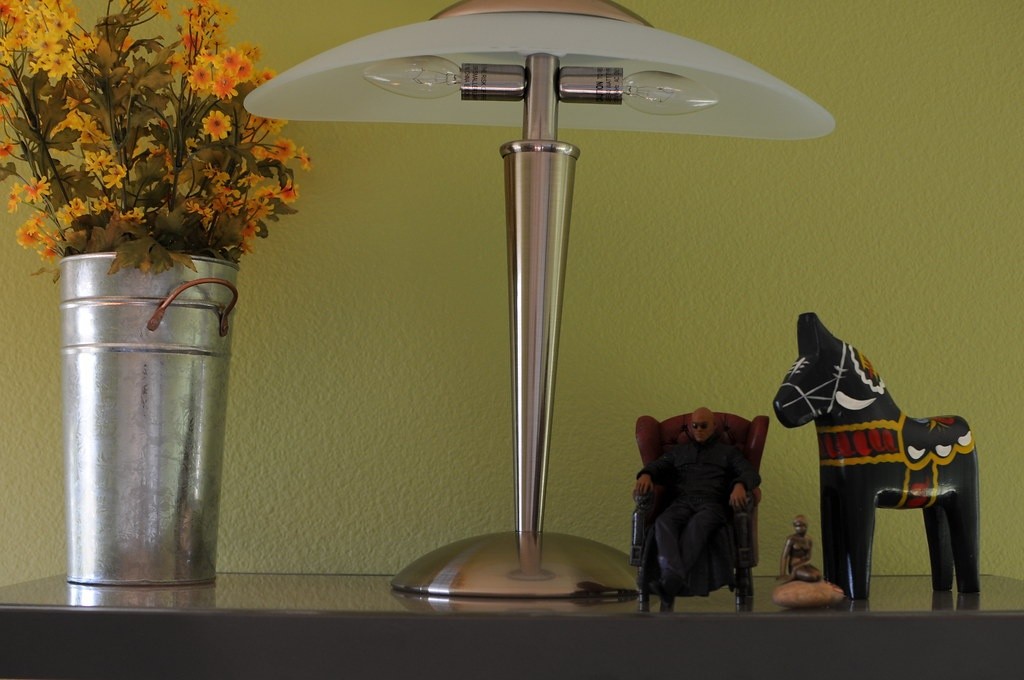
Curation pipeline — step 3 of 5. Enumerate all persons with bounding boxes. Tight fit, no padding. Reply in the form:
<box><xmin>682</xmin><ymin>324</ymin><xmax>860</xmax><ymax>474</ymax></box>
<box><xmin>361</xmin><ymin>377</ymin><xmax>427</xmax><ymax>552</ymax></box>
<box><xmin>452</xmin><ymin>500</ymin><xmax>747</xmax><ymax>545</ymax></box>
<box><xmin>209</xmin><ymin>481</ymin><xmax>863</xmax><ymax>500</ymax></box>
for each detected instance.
<box><xmin>634</xmin><ymin>408</ymin><xmax>761</xmax><ymax>613</ymax></box>
<box><xmin>777</xmin><ymin>514</ymin><xmax>822</xmax><ymax>583</ymax></box>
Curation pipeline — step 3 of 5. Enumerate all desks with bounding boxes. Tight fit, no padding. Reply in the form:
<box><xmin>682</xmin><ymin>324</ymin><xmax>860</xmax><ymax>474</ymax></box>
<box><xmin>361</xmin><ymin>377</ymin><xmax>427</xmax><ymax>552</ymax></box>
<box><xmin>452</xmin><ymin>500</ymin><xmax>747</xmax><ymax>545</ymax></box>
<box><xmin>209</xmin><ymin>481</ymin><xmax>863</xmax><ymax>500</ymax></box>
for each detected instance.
<box><xmin>0</xmin><ymin>573</ymin><xmax>1024</xmax><ymax>680</ymax></box>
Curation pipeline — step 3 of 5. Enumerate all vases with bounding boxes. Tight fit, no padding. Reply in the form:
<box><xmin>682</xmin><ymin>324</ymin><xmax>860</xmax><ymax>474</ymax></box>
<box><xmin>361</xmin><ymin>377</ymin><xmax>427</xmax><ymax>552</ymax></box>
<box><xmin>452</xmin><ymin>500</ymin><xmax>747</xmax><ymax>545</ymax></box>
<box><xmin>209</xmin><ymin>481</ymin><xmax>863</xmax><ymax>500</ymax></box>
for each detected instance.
<box><xmin>61</xmin><ymin>253</ymin><xmax>242</xmax><ymax>588</ymax></box>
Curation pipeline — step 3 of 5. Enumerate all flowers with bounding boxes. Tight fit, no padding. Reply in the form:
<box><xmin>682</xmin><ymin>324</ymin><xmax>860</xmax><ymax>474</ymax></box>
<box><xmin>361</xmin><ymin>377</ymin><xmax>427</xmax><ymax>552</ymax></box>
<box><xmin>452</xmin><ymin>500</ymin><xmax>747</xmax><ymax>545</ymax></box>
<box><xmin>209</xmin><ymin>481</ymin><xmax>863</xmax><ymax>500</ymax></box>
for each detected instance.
<box><xmin>0</xmin><ymin>0</ymin><xmax>314</xmax><ymax>278</ymax></box>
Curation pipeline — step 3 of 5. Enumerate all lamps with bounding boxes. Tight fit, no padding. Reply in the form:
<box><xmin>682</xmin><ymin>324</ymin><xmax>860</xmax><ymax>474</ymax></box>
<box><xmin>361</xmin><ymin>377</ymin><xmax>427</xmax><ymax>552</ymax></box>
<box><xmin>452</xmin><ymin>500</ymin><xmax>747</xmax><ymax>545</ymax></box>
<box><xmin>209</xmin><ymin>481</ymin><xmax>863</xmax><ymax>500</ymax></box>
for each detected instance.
<box><xmin>239</xmin><ymin>0</ymin><xmax>839</xmax><ymax>599</ymax></box>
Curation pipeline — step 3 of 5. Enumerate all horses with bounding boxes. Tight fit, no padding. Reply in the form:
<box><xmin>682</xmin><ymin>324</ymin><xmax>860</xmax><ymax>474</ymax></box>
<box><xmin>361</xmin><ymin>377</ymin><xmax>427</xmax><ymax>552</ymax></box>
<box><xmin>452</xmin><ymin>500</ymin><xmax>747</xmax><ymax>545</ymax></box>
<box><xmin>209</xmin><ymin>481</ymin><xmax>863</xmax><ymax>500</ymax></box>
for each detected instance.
<box><xmin>773</xmin><ymin>313</ymin><xmax>979</xmax><ymax>598</ymax></box>
